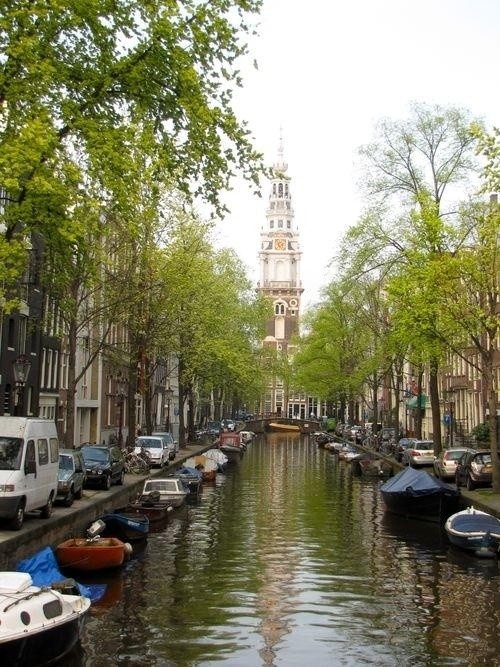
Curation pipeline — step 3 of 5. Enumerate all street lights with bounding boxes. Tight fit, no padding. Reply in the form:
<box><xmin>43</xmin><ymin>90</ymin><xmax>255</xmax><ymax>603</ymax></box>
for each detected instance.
<box><xmin>13</xmin><ymin>352</ymin><xmax>32</xmax><ymax>416</ymax></box>
<box><xmin>379</xmin><ymin>397</ymin><xmax>385</xmax><ymax>430</ymax></box>
<box><xmin>446</xmin><ymin>387</ymin><xmax>457</xmax><ymax>447</ymax></box>
<box><xmin>403</xmin><ymin>392</ymin><xmax>411</xmax><ymax>437</ymax></box>
<box><xmin>166</xmin><ymin>387</ymin><xmax>174</xmax><ymax>432</ymax></box>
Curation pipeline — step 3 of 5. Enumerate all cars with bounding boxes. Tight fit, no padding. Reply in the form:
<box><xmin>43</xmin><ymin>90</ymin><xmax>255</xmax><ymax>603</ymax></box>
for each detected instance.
<box><xmin>335</xmin><ymin>422</ymin><xmax>436</xmax><ymax>466</ymax></box>
<box><xmin>208</xmin><ymin>419</ymin><xmax>235</xmax><ymax>436</ymax></box>
<box><xmin>135</xmin><ymin>432</ymin><xmax>177</xmax><ymax>468</ymax></box>
<box><xmin>434</xmin><ymin>446</ymin><xmax>492</xmax><ymax>490</ymax></box>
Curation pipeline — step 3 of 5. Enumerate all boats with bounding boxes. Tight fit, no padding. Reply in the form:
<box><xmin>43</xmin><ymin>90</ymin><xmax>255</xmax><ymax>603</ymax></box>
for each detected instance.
<box><xmin>445</xmin><ymin>505</ymin><xmax>500</xmax><ymax>558</ymax></box>
<box><xmin>316</xmin><ymin>434</ymin><xmax>392</xmax><ymax>476</ymax></box>
<box><xmin>182</xmin><ymin>431</ymin><xmax>254</xmax><ymax>481</ymax></box>
<box><xmin>380</xmin><ymin>467</ymin><xmax>459</xmax><ymax>522</ymax></box>
<box><xmin>56</xmin><ymin>467</ymin><xmax>201</xmax><ymax>578</ymax></box>
<box><xmin>0</xmin><ymin>571</ymin><xmax>92</xmax><ymax>667</ymax></box>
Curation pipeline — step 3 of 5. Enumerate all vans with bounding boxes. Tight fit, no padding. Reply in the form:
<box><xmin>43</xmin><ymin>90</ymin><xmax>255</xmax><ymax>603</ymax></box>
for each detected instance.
<box><xmin>0</xmin><ymin>416</ymin><xmax>59</xmax><ymax>530</ymax></box>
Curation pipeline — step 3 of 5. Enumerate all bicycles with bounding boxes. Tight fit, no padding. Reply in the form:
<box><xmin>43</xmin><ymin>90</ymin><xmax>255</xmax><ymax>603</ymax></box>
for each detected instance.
<box><xmin>120</xmin><ymin>445</ymin><xmax>152</xmax><ymax>475</ymax></box>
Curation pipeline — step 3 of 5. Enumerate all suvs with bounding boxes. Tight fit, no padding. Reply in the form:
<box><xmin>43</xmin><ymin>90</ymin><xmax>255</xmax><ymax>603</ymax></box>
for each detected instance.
<box><xmin>80</xmin><ymin>442</ymin><xmax>126</xmax><ymax>490</ymax></box>
<box><xmin>57</xmin><ymin>449</ymin><xmax>86</xmax><ymax>507</ymax></box>
